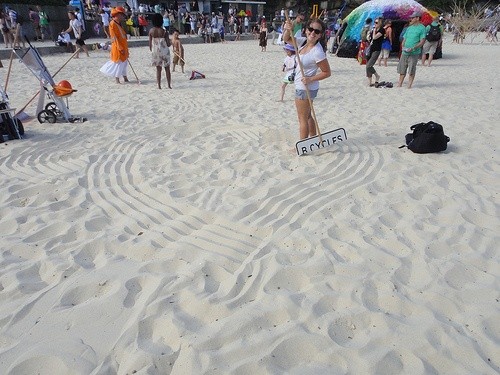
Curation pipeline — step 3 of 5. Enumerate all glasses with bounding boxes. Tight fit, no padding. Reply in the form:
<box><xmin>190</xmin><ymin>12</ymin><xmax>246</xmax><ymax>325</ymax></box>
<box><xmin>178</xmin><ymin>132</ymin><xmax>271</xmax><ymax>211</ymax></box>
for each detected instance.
<box><xmin>298</xmin><ymin>18</ymin><xmax>304</xmax><ymax>21</ymax></box>
<box><xmin>373</xmin><ymin>21</ymin><xmax>378</xmax><ymax>24</ymax></box>
<box><xmin>307</xmin><ymin>27</ymin><xmax>320</xmax><ymax>34</ymax></box>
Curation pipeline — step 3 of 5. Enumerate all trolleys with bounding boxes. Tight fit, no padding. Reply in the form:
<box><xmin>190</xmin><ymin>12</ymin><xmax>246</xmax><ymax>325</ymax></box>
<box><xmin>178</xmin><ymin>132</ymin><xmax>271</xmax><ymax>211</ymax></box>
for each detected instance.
<box><xmin>13</xmin><ymin>30</ymin><xmax>87</xmax><ymax>126</ymax></box>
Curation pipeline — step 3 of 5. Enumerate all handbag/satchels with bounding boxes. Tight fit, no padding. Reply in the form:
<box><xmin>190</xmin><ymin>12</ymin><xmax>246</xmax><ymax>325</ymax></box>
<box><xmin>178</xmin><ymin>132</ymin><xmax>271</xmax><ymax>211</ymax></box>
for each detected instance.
<box><xmin>3</xmin><ymin>27</ymin><xmax>9</xmax><ymax>32</ymax></box>
<box><xmin>406</xmin><ymin>121</ymin><xmax>450</xmax><ymax>153</ymax></box>
<box><xmin>365</xmin><ymin>47</ymin><xmax>371</xmax><ymax>61</ymax></box>
<box><xmin>81</xmin><ymin>30</ymin><xmax>89</xmax><ymax>40</ymax></box>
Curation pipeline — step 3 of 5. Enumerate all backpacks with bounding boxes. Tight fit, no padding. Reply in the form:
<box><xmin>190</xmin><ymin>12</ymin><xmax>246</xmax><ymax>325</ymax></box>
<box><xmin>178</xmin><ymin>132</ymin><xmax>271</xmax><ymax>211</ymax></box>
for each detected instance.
<box><xmin>44</xmin><ymin>11</ymin><xmax>50</xmax><ymax>23</ymax></box>
<box><xmin>12</xmin><ymin>11</ymin><xmax>23</xmax><ymax>26</ymax></box>
<box><xmin>426</xmin><ymin>24</ymin><xmax>441</xmax><ymax>41</ymax></box>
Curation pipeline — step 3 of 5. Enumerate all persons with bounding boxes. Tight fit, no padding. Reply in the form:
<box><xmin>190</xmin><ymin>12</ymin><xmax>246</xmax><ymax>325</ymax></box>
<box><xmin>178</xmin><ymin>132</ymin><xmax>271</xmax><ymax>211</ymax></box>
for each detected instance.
<box><xmin>57</xmin><ymin>29</ymin><xmax>73</xmax><ymax>52</ymax></box>
<box><xmin>149</xmin><ymin>13</ymin><xmax>173</xmax><ymax>89</ymax></box>
<box><xmin>421</xmin><ymin>16</ymin><xmax>443</xmax><ymax>66</ymax></box>
<box><xmin>484</xmin><ymin>4</ymin><xmax>500</xmax><ymax>36</ymax></box>
<box><xmin>99</xmin><ymin>7</ymin><xmax>129</xmax><ymax>83</ymax></box>
<box><xmin>399</xmin><ymin>23</ymin><xmax>410</xmax><ymax>59</ymax></box>
<box><xmin>170</xmin><ymin>29</ymin><xmax>184</xmax><ymax>72</ymax></box>
<box><xmin>439</xmin><ymin>12</ymin><xmax>459</xmax><ymax>32</ymax></box>
<box><xmin>277</xmin><ymin>43</ymin><xmax>296</xmax><ymax>102</ymax></box>
<box><xmin>0</xmin><ymin>8</ymin><xmax>26</xmax><ymax>48</ymax></box>
<box><xmin>377</xmin><ymin>20</ymin><xmax>392</xmax><ymax>67</ymax></box>
<box><xmin>183</xmin><ymin>5</ymin><xmax>250</xmax><ymax>44</ymax></box>
<box><xmin>366</xmin><ymin>18</ymin><xmax>386</xmax><ymax>86</ymax></box>
<box><xmin>282</xmin><ymin>18</ymin><xmax>331</xmax><ymax>140</ymax></box>
<box><xmin>396</xmin><ymin>11</ymin><xmax>427</xmax><ymax>89</ymax></box>
<box><xmin>75</xmin><ymin>3</ymin><xmax>180</xmax><ymax>38</ymax></box>
<box><xmin>28</xmin><ymin>5</ymin><xmax>51</xmax><ymax>42</ymax></box>
<box><xmin>253</xmin><ymin>8</ymin><xmax>343</xmax><ymax>53</ymax></box>
<box><xmin>362</xmin><ymin>18</ymin><xmax>372</xmax><ymax>53</ymax></box>
<box><xmin>61</xmin><ymin>11</ymin><xmax>89</xmax><ymax>59</ymax></box>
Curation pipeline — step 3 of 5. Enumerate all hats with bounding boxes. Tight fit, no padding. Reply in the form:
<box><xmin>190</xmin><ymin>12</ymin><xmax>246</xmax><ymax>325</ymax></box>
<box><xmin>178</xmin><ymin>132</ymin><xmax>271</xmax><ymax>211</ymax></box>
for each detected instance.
<box><xmin>116</xmin><ymin>7</ymin><xmax>127</xmax><ymax>15</ymax></box>
<box><xmin>410</xmin><ymin>11</ymin><xmax>421</xmax><ymax>18</ymax></box>
<box><xmin>283</xmin><ymin>44</ymin><xmax>295</xmax><ymax>51</ymax></box>
